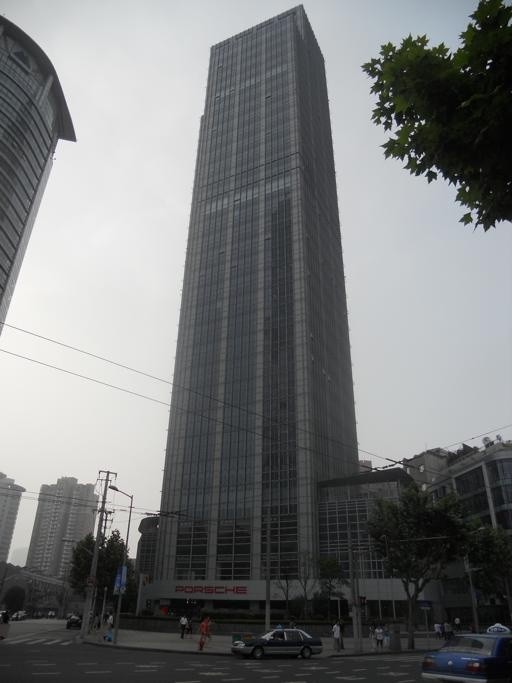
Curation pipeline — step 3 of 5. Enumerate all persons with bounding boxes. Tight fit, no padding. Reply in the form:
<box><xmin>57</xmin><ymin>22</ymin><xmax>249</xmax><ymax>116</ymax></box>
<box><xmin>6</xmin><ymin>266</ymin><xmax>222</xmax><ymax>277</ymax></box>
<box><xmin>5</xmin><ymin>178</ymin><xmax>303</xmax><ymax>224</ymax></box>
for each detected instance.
<box><xmin>368</xmin><ymin>623</ymin><xmax>390</xmax><ymax>649</ymax></box>
<box><xmin>87</xmin><ymin>610</ymin><xmax>114</xmax><ymax>641</ymax></box>
<box><xmin>436</xmin><ymin>617</ymin><xmax>461</xmax><ymax>640</ymax></box>
<box><xmin>199</xmin><ymin>615</ymin><xmax>215</xmax><ymax>649</ymax></box>
<box><xmin>332</xmin><ymin>617</ymin><xmax>345</xmax><ymax>651</ymax></box>
<box><xmin>179</xmin><ymin>615</ymin><xmax>193</xmax><ymax>639</ymax></box>
<box><xmin>276</xmin><ymin>623</ymin><xmax>296</xmax><ymax>629</ymax></box>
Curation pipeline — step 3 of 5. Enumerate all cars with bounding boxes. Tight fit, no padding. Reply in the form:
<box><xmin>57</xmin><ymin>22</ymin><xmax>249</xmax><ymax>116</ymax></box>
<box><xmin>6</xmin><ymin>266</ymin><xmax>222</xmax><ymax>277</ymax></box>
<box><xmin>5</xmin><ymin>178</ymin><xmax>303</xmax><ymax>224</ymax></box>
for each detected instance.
<box><xmin>12</xmin><ymin>611</ymin><xmax>28</xmax><ymax>621</ymax></box>
<box><xmin>422</xmin><ymin>623</ymin><xmax>512</xmax><ymax>683</ymax></box>
<box><xmin>231</xmin><ymin>629</ymin><xmax>323</xmax><ymax>658</ymax></box>
<box><xmin>48</xmin><ymin>611</ymin><xmax>56</xmax><ymax>618</ymax></box>
<box><xmin>65</xmin><ymin>612</ymin><xmax>81</xmax><ymax>629</ymax></box>
<box><xmin>33</xmin><ymin>612</ymin><xmax>43</xmax><ymax>619</ymax></box>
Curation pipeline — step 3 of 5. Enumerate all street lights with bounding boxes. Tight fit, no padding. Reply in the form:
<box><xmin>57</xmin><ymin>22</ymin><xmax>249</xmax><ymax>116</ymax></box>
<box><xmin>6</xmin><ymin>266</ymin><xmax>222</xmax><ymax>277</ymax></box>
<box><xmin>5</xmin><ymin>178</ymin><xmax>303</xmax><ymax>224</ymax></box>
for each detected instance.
<box><xmin>109</xmin><ymin>486</ymin><xmax>132</xmax><ymax>644</ymax></box>
<box><xmin>61</xmin><ymin>538</ymin><xmax>98</xmax><ymax>640</ymax></box>
